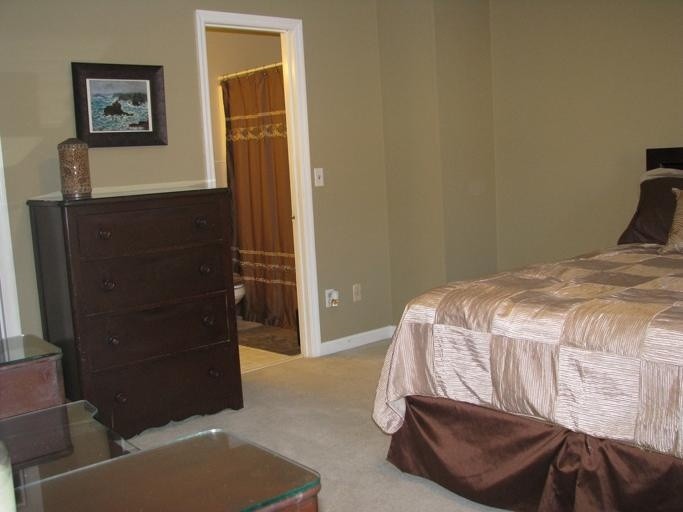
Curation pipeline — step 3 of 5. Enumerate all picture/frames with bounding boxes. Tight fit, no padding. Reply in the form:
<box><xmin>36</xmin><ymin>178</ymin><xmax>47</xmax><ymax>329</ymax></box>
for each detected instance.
<box><xmin>72</xmin><ymin>62</ymin><xmax>168</xmax><ymax>148</ymax></box>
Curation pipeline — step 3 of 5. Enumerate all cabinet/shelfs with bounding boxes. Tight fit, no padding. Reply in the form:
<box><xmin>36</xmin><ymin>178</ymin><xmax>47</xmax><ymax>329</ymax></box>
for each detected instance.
<box><xmin>25</xmin><ymin>183</ymin><xmax>242</xmax><ymax>436</ymax></box>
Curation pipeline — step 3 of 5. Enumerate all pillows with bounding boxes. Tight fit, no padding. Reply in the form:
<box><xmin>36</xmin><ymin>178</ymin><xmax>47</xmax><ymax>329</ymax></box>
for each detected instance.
<box><xmin>615</xmin><ymin>177</ymin><xmax>683</xmax><ymax>254</ymax></box>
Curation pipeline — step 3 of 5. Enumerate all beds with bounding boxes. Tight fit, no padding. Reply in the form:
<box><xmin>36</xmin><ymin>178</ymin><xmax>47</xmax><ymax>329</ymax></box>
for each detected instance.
<box><xmin>400</xmin><ymin>148</ymin><xmax>683</xmax><ymax>512</ymax></box>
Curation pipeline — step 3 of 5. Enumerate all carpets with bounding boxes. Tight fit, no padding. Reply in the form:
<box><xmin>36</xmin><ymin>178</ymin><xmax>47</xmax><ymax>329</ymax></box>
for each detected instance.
<box><xmin>236</xmin><ymin>324</ymin><xmax>301</xmax><ymax>356</ymax></box>
